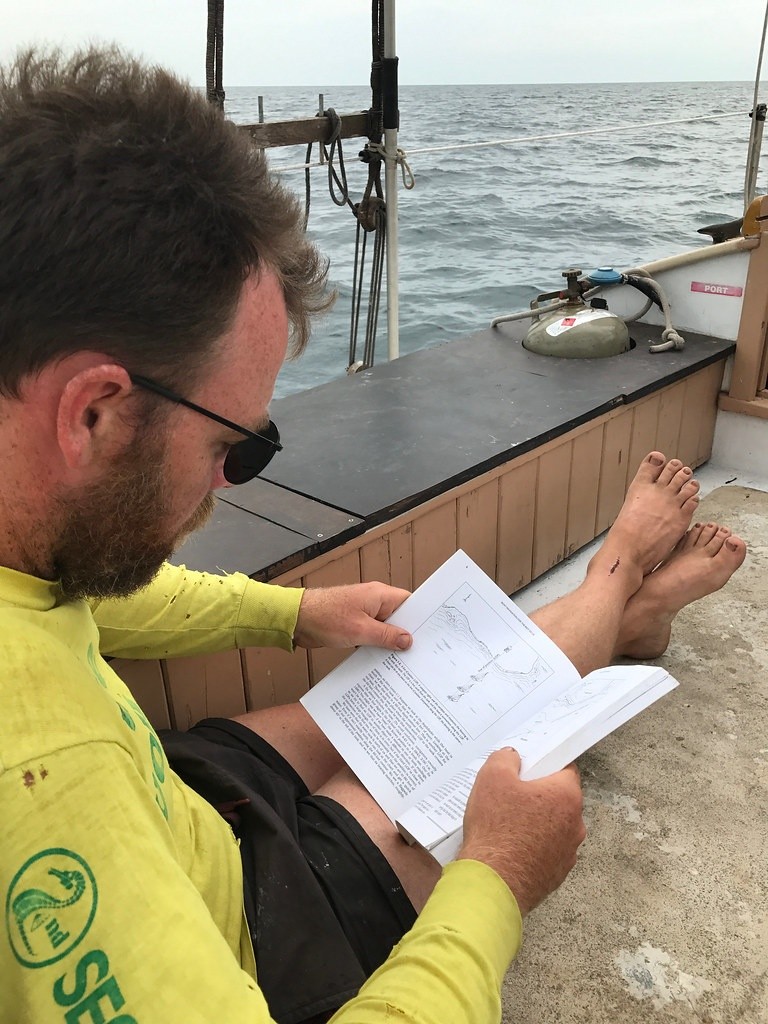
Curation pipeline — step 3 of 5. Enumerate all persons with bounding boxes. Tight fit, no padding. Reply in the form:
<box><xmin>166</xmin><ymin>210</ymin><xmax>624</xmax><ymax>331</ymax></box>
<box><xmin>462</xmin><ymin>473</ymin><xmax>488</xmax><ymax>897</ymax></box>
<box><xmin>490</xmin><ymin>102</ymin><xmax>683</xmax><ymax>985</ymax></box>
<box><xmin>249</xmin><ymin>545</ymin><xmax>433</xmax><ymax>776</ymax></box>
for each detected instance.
<box><xmin>0</xmin><ymin>47</ymin><xmax>747</xmax><ymax>1024</ymax></box>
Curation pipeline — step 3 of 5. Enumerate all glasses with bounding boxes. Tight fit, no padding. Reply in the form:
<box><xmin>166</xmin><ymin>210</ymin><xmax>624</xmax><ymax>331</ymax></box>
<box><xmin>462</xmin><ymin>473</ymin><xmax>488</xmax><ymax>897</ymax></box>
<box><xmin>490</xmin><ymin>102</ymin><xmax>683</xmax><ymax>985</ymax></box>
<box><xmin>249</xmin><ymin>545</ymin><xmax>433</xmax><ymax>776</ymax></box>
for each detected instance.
<box><xmin>129</xmin><ymin>374</ymin><xmax>283</xmax><ymax>485</ymax></box>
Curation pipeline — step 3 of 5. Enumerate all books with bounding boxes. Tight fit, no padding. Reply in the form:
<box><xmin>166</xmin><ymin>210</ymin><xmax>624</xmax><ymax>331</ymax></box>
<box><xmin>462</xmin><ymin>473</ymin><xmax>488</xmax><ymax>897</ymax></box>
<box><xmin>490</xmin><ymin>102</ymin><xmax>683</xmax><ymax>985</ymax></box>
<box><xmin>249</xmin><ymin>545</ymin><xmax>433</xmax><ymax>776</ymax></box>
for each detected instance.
<box><xmin>300</xmin><ymin>548</ymin><xmax>680</xmax><ymax>870</ymax></box>
